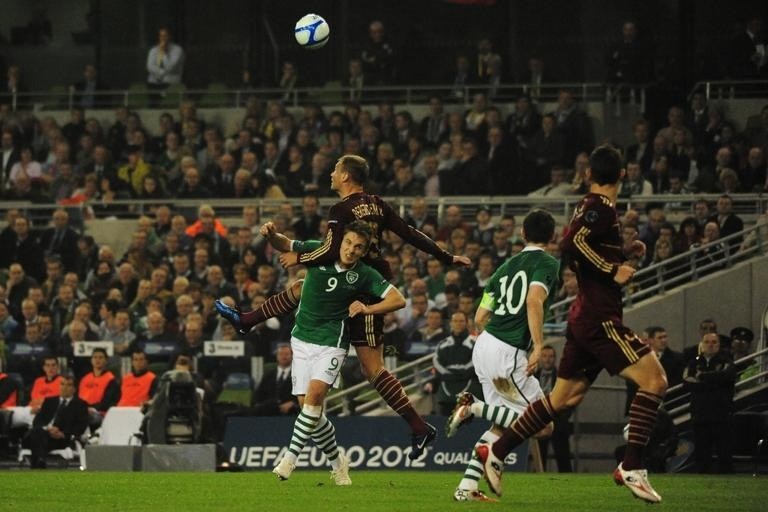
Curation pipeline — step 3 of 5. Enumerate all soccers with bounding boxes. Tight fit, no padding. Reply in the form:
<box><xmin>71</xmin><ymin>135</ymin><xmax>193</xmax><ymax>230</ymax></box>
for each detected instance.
<box><xmin>296</xmin><ymin>14</ymin><xmax>330</xmax><ymax>49</ymax></box>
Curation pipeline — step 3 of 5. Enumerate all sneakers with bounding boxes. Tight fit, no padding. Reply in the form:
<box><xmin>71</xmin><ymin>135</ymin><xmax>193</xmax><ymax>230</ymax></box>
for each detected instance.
<box><xmin>444</xmin><ymin>392</ymin><xmax>477</xmax><ymax>439</ymax></box>
<box><xmin>331</xmin><ymin>455</ymin><xmax>352</xmax><ymax>485</ymax></box>
<box><xmin>215</xmin><ymin>300</ymin><xmax>250</xmax><ymax>336</ymax></box>
<box><xmin>613</xmin><ymin>463</ymin><xmax>662</xmax><ymax>503</ymax></box>
<box><xmin>475</xmin><ymin>442</ymin><xmax>505</xmax><ymax>497</ymax></box>
<box><xmin>273</xmin><ymin>451</ymin><xmax>297</xmax><ymax>480</ymax></box>
<box><xmin>454</xmin><ymin>488</ymin><xmax>498</xmax><ymax>503</ymax></box>
<box><xmin>409</xmin><ymin>423</ymin><xmax>436</xmax><ymax>460</ymax></box>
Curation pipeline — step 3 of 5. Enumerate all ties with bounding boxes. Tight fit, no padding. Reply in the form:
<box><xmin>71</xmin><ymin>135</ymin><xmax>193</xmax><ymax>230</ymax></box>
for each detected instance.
<box><xmin>53</xmin><ymin>399</ymin><xmax>66</xmax><ymax>425</ymax></box>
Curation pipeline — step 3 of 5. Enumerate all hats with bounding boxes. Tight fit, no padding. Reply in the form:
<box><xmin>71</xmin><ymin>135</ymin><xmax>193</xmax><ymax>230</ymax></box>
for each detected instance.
<box><xmin>730</xmin><ymin>327</ymin><xmax>754</xmax><ymax>343</ymax></box>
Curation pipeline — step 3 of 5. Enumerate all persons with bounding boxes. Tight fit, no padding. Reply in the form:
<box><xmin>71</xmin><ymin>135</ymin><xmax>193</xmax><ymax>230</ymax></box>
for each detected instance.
<box><xmin>214</xmin><ymin>155</ymin><xmax>471</xmax><ymax>463</ymax></box>
<box><xmin>626</xmin><ymin>327</ymin><xmax>685</xmax><ymax>415</ymax></box>
<box><xmin>432</xmin><ymin>312</ymin><xmax>482</xmax><ymax>418</ymax></box>
<box><xmin>384</xmin><ymin>199</ymin><xmax>574</xmax><ymax>357</ymax></box>
<box><xmin>445</xmin><ymin>209</ymin><xmax>560</xmax><ymax>502</ymax></box>
<box><xmin>730</xmin><ymin>327</ymin><xmax>757</xmax><ymax>371</ymax></box>
<box><xmin>643</xmin><ymin>327</ymin><xmax>684</xmax><ymax>363</ymax></box>
<box><xmin>476</xmin><ymin>143</ymin><xmax>668</xmax><ymax>504</ymax></box>
<box><xmin>1</xmin><ymin>2</ymin><xmax>768</xmax><ymax>201</ymax></box>
<box><xmin>260</xmin><ymin>221</ymin><xmax>406</xmax><ymax>486</ymax></box>
<box><xmin>534</xmin><ymin>344</ymin><xmax>576</xmax><ymax>473</ymax></box>
<box><xmin>682</xmin><ymin>319</ymin><xmax>731</xmax><ymax>368</ymax></box>
<box><xmin>683</xmin><ymin>333</ymin><xmax>737</xmax><ymax>435</ymax></box>
<box><xmin>1</xmin><ymin>201</ymin><xmax>335</xmax><ymax>469</ymax></box>
<box><xmin>623</xmin><ymin>195</ymin><xmax>752</xmax><ymax>293</ymax></box>
